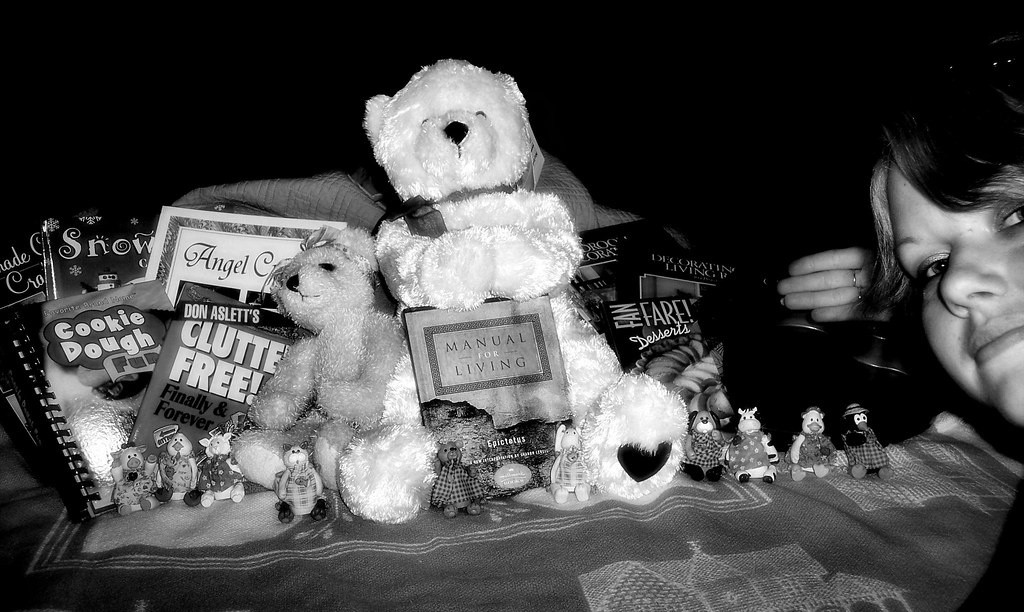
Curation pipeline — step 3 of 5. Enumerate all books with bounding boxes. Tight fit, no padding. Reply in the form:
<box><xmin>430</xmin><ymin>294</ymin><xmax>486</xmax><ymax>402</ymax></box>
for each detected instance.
<box><xmin>573</xmin><ymin>216</ymin><xmax>739</xmax><ymax>376</ymax></box>
<box><xmin>0</xmin><ymin>206</ymin><xmax>350</xmax><ymax>530</ymax></box>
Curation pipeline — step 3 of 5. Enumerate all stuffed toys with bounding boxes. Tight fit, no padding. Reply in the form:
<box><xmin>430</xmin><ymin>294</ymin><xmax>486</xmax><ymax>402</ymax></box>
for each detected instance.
<box><xmin>80</xmin><ymin>58</ymin><xmax>891</xmax><ymax>522</ymax></box>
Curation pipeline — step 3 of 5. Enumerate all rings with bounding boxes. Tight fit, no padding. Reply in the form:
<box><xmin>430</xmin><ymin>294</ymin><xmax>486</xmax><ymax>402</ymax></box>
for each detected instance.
<box><xmin>850</xmin><ymin>268</ymin><xmax>859</xmax><ymax>289</ymax></box>
<box><xmin>856</xmin><ymin>288</ymin><xmax>862</xmax><ymax>301</ymax></box>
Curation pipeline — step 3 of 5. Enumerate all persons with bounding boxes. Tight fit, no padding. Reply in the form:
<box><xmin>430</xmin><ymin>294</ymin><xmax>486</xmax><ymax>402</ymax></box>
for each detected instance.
<box><xmin>778</xmin><ymin>32</ymin><xmax>1024</xmax><ymax>610</ymax></box>
